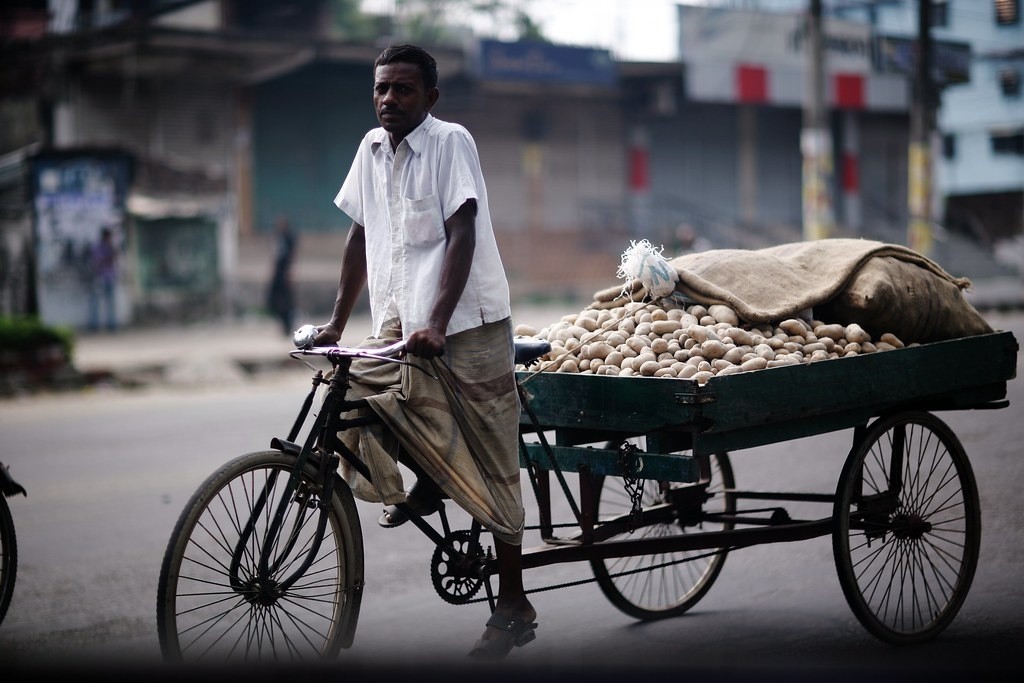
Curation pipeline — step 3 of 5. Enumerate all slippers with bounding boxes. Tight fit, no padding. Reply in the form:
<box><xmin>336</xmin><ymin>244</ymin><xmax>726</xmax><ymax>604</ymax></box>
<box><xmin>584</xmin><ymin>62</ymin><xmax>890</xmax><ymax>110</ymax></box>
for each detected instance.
<box><xmin>466</xmin><ymin>616</ymin><xmax>538</xmax><ymax>665</ymax></box>
<box><xmin>376</xmin><ymin>486</ymin><xmax>442</xmax><ymax>528</ymax></box>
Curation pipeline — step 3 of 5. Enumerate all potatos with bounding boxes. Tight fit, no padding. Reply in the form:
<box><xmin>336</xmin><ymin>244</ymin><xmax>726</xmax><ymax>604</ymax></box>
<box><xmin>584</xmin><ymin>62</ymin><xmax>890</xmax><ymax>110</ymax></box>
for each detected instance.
<box><xmin>515</xmin><ymin>304</ymin><xmax>919</xmax><ymax>382</ymax></box>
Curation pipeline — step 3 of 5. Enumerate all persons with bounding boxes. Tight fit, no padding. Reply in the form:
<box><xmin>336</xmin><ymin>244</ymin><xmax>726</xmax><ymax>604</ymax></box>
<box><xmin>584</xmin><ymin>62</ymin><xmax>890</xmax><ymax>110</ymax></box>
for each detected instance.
<box><xmin>87</xmin><ymin>228</ymin><xmax>120</xmax><ymax>332</ymax></box>
<box><xmin>267</xmin><ymin>216</ymin><xmax>297</xmax><ymax>339</ymax></box>
<box><xmin>309</xmin><ymin>43</ymin><xmax>539</xmax><ymax>664</ymax></box>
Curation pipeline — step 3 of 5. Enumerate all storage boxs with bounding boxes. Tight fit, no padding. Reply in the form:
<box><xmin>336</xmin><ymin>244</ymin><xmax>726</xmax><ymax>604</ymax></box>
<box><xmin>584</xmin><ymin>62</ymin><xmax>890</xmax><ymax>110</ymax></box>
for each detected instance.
<box><xmin>514</xmin><ymin>327</ymin><xmax>1020</xmax><ymax>435</ymax></box>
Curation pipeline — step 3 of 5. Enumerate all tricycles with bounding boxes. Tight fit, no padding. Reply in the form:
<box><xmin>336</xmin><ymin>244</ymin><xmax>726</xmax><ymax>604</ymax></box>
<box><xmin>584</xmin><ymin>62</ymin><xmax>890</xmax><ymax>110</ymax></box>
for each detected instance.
<box><xmin>156</xmin><ymin>330</ymin><xmax>1020</xmax><ymax>670</ymax></box>
